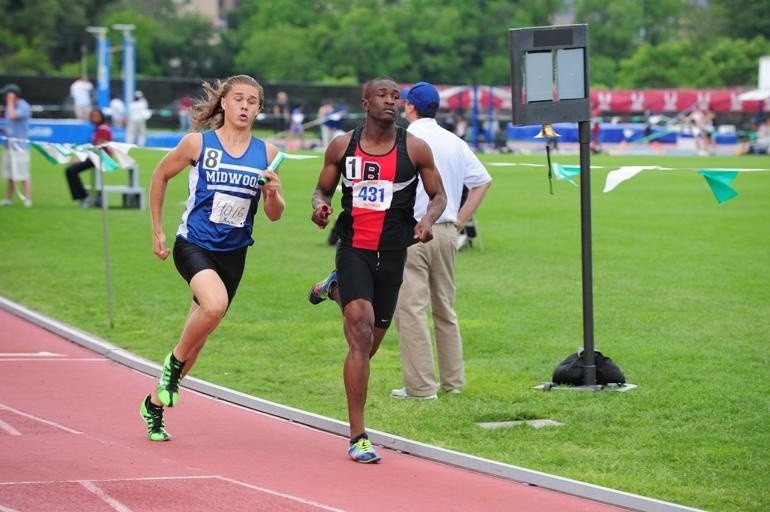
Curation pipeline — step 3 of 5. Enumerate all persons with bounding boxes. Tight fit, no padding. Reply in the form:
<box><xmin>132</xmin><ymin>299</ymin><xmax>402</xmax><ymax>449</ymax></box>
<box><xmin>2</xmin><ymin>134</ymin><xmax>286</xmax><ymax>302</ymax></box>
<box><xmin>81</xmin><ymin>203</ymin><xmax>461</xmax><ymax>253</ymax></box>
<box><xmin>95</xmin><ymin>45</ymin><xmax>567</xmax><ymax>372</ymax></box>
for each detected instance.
<box><xmin>389</xmin><ymin>81</ymin><xmax>493</xmax><ymax>401</ymax></box>
<box><xmin>137</xmin><ymin>73</ymin><xmax>285</xmax><ymax>442</ymax></box>
<box><xmin>308</xmin><ymin>77</ymin><xmax>449</xmax><ymax>463</ymax></box>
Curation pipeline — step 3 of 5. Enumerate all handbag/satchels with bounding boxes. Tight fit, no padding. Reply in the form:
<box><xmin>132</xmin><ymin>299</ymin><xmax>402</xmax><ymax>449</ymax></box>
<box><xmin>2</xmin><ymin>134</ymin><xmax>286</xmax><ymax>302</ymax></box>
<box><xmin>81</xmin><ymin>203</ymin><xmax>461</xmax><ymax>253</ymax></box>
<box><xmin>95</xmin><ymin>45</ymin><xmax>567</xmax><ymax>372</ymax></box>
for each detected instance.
<box><xmin>550</xmin><ymin>346</ymin><xmax>627</xmax><ymax>385</ymax></box>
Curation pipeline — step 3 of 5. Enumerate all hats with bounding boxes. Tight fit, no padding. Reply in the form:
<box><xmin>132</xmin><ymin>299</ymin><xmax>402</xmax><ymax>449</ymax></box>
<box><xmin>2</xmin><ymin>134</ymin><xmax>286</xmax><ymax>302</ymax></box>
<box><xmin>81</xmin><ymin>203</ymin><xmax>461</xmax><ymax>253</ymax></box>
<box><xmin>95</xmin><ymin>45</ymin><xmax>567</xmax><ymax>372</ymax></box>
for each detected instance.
<box><xmin>0</xmin><ymin>82</ymin><xmax>22</xmax><ymax>95</ymax></box>
<box><xmin>399</xmin><ymin>81</ymin><xmax>440</xmax><ymax>114</ymax></box>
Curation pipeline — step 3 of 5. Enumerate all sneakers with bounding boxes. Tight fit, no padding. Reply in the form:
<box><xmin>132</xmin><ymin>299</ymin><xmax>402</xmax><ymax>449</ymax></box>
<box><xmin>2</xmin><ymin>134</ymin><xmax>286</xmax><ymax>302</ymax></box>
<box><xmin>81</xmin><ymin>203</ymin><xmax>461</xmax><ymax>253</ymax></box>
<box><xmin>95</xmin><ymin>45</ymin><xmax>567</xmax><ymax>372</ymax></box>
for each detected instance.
<box><xmin>156</xmin><ymin>351</ymin><xmax>189</xmax><ymax>409</ymax></box>
<box><xmin>139</xmin><ymin>393</ymin><xmax>173</xmax><ymax>443</ymax></box>
<box><xmin>390</xmin><ymin>386</ymin><xmax>437</xmax><ymax>400</ymax></box>
<box><xmin>0</xmin><ymin>198</ymin><xmax>33</xmax><ymax>208</ymax></box>
<box><xmin>348</xmin><ymin>436</ymin><xmax>382</xmax><ymax>464</ymax></box>
<box><xmin>308</xmin><ymin>268</ymin><xmax>339</xmax><ymax>306</ymax></box>
<box><xmin>77</xmin><ymin>195</ymin><xmax>96</xmax><ymax>209</ymax></box>
<box><xmin>436</xmin><ymin>382</ymin><xmax>462</xmax><ymax>394</ymax></box>
